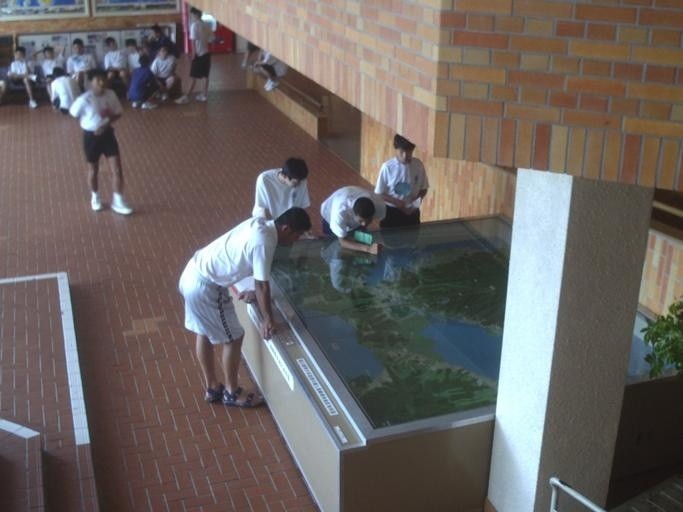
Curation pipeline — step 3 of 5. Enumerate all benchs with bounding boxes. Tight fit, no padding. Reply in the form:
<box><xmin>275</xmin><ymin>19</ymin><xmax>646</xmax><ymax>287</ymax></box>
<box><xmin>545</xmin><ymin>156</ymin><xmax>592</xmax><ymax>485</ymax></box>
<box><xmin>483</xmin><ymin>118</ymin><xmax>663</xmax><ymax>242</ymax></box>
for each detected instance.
<box><xmin>0</xmin><ymin>63</ymin><xmax>181</xmax><ymax>97</ymax></box>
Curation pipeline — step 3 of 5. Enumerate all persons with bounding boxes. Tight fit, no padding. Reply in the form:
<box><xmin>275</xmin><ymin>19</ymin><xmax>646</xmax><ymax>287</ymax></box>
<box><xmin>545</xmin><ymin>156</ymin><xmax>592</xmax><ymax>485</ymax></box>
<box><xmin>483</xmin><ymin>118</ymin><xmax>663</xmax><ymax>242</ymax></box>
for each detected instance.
<box><xmin>381</xmin><ymin>231</ymin><xmax>424</xmax><ymax>319</ymax></box>
<box><xmin>1</xmin><ymin>23</ymin><xmax>286</xmax><ymax>116</ymax></box>
<box><xmin>250</xmin><ymin>158</ymin><xmax>311</xmax><ymax>239</ymax></box>
<box><xmin>173</xmin><ymin>7</ymin><xmax>213</xmax><ymax>107</ymax></box>
<box><xmin>374</xmin><ymin>132</ymin><xmax>429</xmax><ymax>229</ymax></box>
<box><xmin>318</xmin><ymin>184</ymin><xmax>387</xmax><ymax>257</ymax></box>
<box><xmin>175</xmin><ymin>206</ymin><xmax>311</xmax><ymax>410</ymax></box>
<box><xmin>68</xmin><ymin>67</ymin><xmax>133</xmax><ymax>218</ymax></box>
<box><xmin>325</xmin><ymin>238</ymin><xmax>374</xmax><ymax>299</ymax></box>
<box><xmin>274</xmin><ymin>246</ymin><xmax>310</xmax><ymax>309</ymax></box>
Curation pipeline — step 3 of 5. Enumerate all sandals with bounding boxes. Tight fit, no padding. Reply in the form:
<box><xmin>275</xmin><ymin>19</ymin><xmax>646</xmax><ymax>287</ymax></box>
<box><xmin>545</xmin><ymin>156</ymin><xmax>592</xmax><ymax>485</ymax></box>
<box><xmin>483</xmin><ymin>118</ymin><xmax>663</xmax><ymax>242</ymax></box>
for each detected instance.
<box><xmin>221</xmin><ymin>386</ymin><xmax>265</xmax><ymax>408</ymax></box>
<box><xmin>203</xmin><ymin>382</ymin><xmax>224</xmax><ymax>402</ymax></box>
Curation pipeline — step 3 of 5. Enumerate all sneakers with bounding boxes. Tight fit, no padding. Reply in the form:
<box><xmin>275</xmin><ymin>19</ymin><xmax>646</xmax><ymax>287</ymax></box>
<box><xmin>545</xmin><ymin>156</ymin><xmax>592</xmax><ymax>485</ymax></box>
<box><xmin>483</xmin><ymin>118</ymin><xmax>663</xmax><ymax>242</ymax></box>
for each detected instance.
<box><xmin>28</xmin><ymin>99</ymin><xmax>38</xmax><ymax>108</ymax></box>
<box><xmin>140</xmin><ymin>101</ymin><xmax>159</xmax><ymax>109</ymax></box>
<box><xmin>109</xmin><ymin>191</ymin><xmax>133</xmax><ymax>215</ymax></box>
<box><xmin>173</xmin><ymin>94</ymin><xmax>192</xmax><ymax>104</ymax></box>
<box><xmin>28</xmin><ymin>73</ymin><xmax>39</xmax><ymax>82</ymax></box>
<box><xmin>195</xmin><ymin>93</ymin><xmax>208</xmax><ymax>103</ymax></box>
<box><xmin>264</xmin><ymin>78</ymin><xmax>280</xmax><ymax>92</ymax></box>
<box><xmin>90</xmin><ymin>191</ymin><xmax>104</xmax><ymax>211</ymax></box>
<box><xmin>132</xmin><ymin>100</ymin><xmax>141</xmax><ymax>108</ymax></box>
<box><xmin>161</xmin><ymin>93</ymin><xmax>168</xmax><ymax>100</ymax></box>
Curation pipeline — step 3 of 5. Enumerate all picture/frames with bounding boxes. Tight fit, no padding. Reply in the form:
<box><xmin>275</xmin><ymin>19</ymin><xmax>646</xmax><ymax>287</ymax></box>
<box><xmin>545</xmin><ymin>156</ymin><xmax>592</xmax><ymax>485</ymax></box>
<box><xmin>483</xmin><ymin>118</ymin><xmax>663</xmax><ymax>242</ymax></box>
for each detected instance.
<box><xmin>0</xmin><ymin>0</ymin><xmax>90</xmax><ymax>22</ymax></box>
<box><xmin>91</xmin><ymin>0</ymin><xmax>181</xmax><ymax>18</ymax></box>
